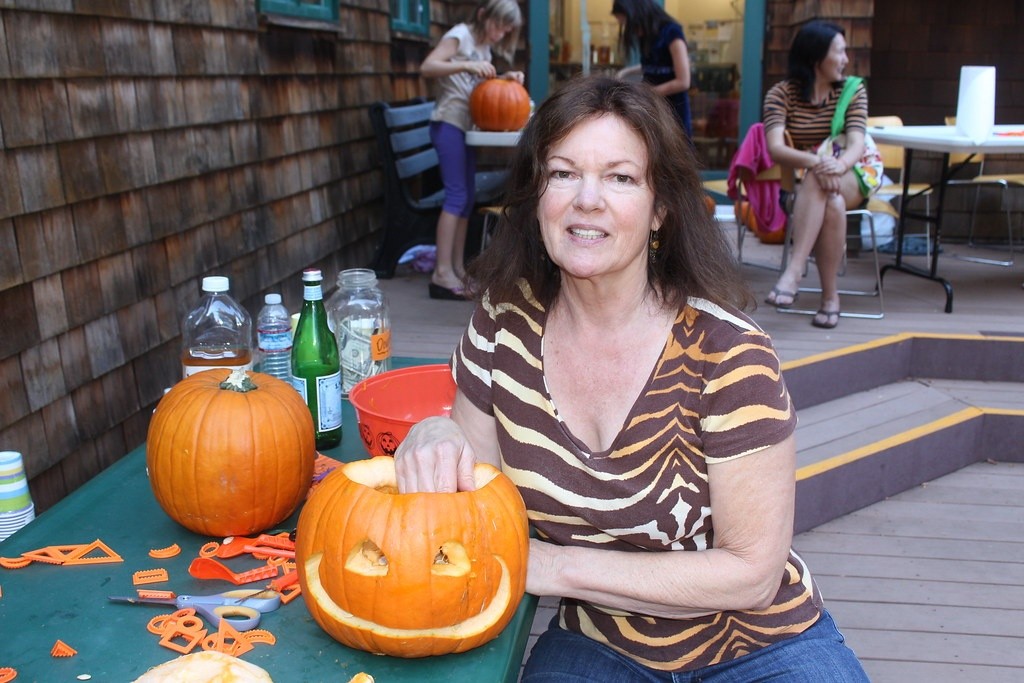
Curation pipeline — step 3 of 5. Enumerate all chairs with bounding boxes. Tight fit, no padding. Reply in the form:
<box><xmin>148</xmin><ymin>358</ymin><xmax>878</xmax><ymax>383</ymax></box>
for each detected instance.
<box><xmin>690</xmin><ymin>100</ymin><xmax>740</xmax><ymax>169</ymax></box>
<box><xmin>737</xmin><ymin>114</ymin><xmax>1024</xmax><ymax>319</ymax></box>
<box><xmin>478</xmin><ymin>206</ymin><xmax>509</xmax><ymax>254</ymax></box>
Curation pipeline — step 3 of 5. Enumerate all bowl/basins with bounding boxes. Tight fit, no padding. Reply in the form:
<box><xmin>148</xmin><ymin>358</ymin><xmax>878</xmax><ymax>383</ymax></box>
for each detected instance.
<box><xmin>349</xmin><ymin>364</ymin><xmax>458</xmax><ymax>458</ymax></box>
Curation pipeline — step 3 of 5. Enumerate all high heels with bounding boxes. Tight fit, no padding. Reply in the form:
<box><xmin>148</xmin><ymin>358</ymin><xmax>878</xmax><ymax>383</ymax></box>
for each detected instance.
<box><xmin>428</xmin><ymin>279</ymin><xmax>481</xmax><ymax>300</ymax></box>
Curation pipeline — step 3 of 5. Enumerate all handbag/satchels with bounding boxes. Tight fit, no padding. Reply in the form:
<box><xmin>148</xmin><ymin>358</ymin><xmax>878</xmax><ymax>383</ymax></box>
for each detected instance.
<box><xmin>817</xmin><ymin>76</ymin><xmax>884</xmax><ymax>197</ymax></box>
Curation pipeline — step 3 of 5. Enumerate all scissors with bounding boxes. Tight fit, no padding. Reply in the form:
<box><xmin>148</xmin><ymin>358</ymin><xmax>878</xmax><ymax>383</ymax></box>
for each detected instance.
<box><xmin>107</xmin><ymin>589</ymin><xmax>281</xmax><ymax>632</ymax></box>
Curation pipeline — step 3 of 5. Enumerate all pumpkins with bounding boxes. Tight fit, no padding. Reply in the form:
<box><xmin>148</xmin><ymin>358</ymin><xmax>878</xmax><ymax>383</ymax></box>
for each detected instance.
<box><xmin>471</xmin><ymin>79</ymin><xmax>531</xmax><ymax>131</ymax></box>
<box><xmin>145</xmin><ymin>367</ymin><xmax>315</xmax><ymax>538</ymax></box>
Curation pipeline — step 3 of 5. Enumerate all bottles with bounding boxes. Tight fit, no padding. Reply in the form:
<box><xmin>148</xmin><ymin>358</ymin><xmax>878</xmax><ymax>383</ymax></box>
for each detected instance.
<box><xmin>330</xmin><ymin>268</ymin><xmax>392</xmax><ymax>401</ymax></box>
<box><xmin>180</xmin><ymin>276</ymin><xmax>255</xmax><ymax>382</ymax></box>
<box><xmin>256</xmin><ymin>293</ymin><xmax>293</xmax><ymax>387</ymax></box>
<box><xmin>291</xmin><ymin>268</ymin><xmax>343</xmax><ymax>450</ymax></box>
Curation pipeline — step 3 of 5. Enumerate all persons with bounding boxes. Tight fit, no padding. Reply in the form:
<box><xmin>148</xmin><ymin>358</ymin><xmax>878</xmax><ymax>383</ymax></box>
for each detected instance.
<box><xmin>763</xmin><ymin>20</ymin><xmax>884</xmax><ymax>329</ymax></box>
<box><xmin>422</xmin><ymin>0</ymin><xmax>524</xmax><ymax>299</ymax></box>
<box><xmin>392</xmin><ymin>73</ymin><xmax>870</xmax><ymax>683</ymax></box>
<box><xmin>613</xmin><ymin>0</ymin><xmax>692</xmax><ymax>148</ymax></box>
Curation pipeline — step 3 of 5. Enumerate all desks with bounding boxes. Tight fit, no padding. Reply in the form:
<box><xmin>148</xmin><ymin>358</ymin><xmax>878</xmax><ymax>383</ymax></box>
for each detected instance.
<box><xmin>867</xmin><ymin>125</ymin><xmax>1024</xmax><ymax>313</ymax></box>
<box><xmin>464</xmin><ymin>112</ymin><xmax>535</xmax><ymax>147</ymax></box>
<box><xmin>0</xmin><ymin>356</ymin><xmax>542</xmax><ymax>683</ymax></box>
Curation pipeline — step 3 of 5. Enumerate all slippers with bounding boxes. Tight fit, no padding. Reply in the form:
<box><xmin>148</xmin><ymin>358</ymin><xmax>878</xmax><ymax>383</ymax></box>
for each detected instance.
<box><xmin>811</xmin><ymin>308</ymin><xmax>841</xmax><ymax>328</ymax></box>
<box><xmin>764</xmin><ymin>284</ymin><xmax>799</xmax><ymax>309</ymax></box>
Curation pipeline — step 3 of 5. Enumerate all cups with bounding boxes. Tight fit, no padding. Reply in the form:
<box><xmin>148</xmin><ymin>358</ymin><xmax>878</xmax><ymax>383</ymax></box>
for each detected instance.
<box><xmin>0</xmin><ymin>450</ymin><xmax>36</xmax><ymax>542</ymax></box>
<box><xmin>291</xmin><ymin>312</ymin><xmax>302</xmax><ymax>341</ymax></box>
<box><xmin>350</xmin><ymin>318</ymin><xmax>385</xmax><ymax>364</ymax></box>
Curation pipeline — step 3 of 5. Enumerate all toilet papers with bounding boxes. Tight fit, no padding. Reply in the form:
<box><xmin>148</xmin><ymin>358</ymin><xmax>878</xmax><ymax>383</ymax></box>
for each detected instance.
<box><xmin>953</xmin><ymin>65</ymin><xmax>997</xmax><ymax>147</ymax></box>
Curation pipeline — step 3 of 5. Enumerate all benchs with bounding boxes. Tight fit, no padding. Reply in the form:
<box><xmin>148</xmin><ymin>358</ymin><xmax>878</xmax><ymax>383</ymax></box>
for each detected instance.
<box><xmin>369</xmin><ymin>93</ymin><xmax>511</xmax><ymax>278</ymax></box>
